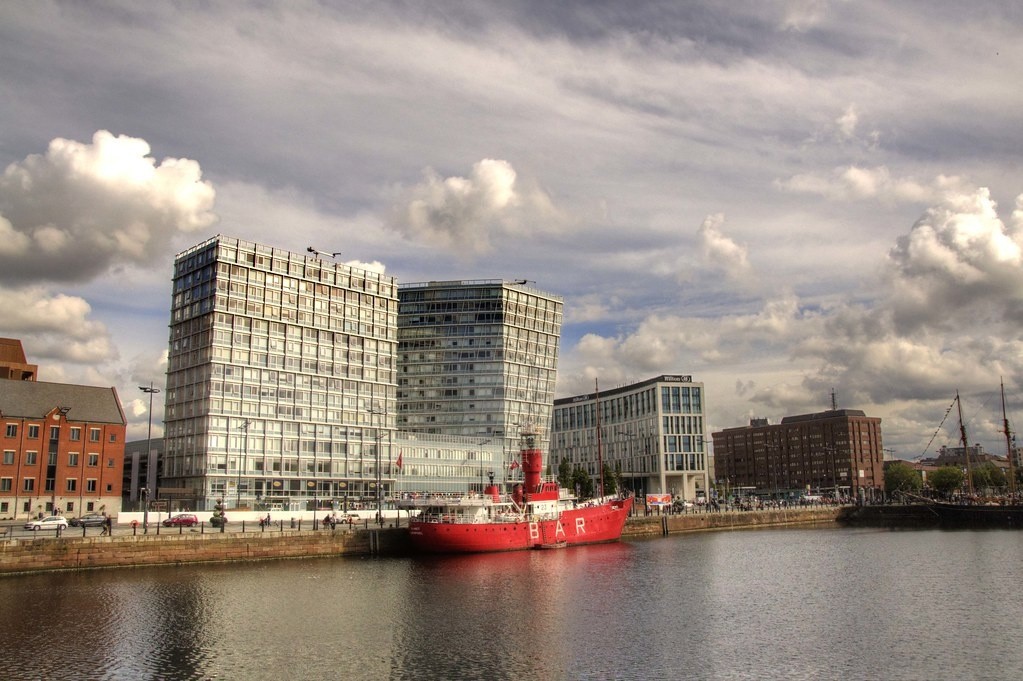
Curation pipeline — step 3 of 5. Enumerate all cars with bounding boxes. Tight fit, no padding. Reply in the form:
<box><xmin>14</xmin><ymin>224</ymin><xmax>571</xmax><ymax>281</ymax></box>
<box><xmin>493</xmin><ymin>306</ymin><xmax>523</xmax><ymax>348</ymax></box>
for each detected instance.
<box><xmin>24</xmin><ymin>515</ymin><xmax>68</xmax><ymax>531</ymax></box>
<box><xmin>163</xmin><ymin>513</ymin><xmax>198</xmax><ymax>528</ymax></box>
<box><xmin>69</xmin><ymin>514</ymin><xmax>107</xmax><ymax>527</ymax></box>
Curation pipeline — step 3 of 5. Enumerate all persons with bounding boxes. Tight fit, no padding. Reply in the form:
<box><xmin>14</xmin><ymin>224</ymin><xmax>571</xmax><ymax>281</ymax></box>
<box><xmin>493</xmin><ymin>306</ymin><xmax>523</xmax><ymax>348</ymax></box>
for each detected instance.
<box><xmin>267</xmin><ymin>513</ymin><xmax>271</xmax><ymax>526</ymax></box>
<box><xmin>324</xmin><ymin>514</ymin><xmax>335</xmax><ymax>530</ymax></box>
<box><xmin>402</xmin><ymin>490</ymin><xmax>429</xmax><ymax>500</ymax></box>
<box><xmin>375</xmin><ymin>512</ymin><xmax>378</xmax><ymax>524</ymax></box>
<box><xmin>100</xmin><ymin>515</ymin><xmax>112</xmax><ymax>536</ymax></box>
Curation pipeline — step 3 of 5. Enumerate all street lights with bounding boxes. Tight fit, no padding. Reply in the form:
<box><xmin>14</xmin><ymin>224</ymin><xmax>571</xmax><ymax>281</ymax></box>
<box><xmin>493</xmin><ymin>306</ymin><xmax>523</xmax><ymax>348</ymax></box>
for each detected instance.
<box><xmin>139</xmin><ymin>381</ymin><xmax>160</xmax><ymax>527</ymax></box>
<box><xmin>618</xmin><ymin>432</ymin><xmax>637</xmax><ymax>514</ymax></box>
<box><xmin>480</xmin><ymin>439</ymin><xmax>492</xmax><ymax>497</ymax></box>
<box><xmin>367</xmin><ymin>406</ymin><xmax>387</xmax><ymax>522</ymax></box>
<box><xmin>238</xmin><ymin>421</ymin><xmax>252</xmax><ymax>507</ymax></box>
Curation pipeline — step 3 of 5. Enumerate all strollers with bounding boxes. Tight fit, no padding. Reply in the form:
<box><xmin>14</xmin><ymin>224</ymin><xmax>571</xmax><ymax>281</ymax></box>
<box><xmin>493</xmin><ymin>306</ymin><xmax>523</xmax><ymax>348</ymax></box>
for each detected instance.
<box><xmin>260</xmin><ymin>518</ymin><xmax>269</xmax><ymax>527</ymax></box>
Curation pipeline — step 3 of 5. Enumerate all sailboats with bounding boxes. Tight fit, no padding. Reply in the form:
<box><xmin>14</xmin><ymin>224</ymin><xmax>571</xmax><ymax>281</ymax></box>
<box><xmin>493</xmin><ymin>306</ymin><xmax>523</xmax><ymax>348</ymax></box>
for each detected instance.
<box><xmin>898</xmin><ymin>375</ymin><xmax>1023</xmax><ymax>528</ymax></box>
<box><xmin>409</xmin><ymin>377</ymin><xmax>635</xmax><ymax>553</ymax></box>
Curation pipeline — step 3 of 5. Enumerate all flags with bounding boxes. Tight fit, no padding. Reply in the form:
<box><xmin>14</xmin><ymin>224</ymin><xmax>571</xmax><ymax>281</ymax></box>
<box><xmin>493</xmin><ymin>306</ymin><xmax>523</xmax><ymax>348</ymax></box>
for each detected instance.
<box><xmin>396</xmin><ymin>453</ymin><xmax>402</xmax><ymax>468</ymax></box>
<box><xmin>510</xmin><ymin>460</ymin><xmax>519</xmax><ymax>470</ymax></box>
